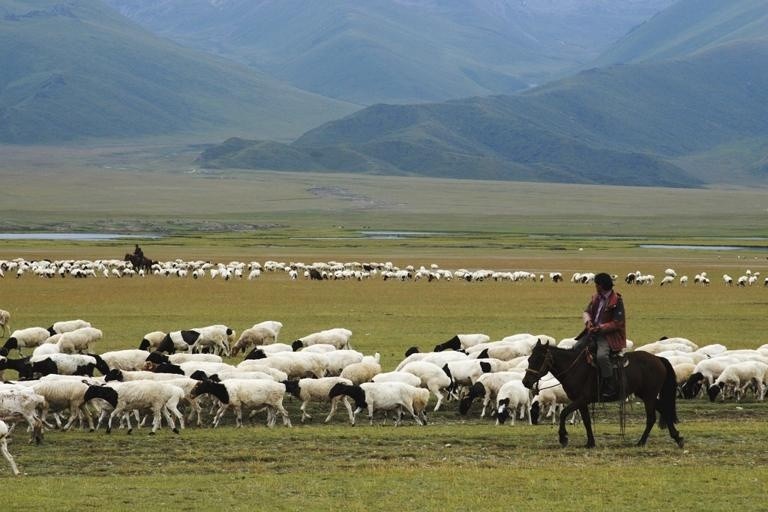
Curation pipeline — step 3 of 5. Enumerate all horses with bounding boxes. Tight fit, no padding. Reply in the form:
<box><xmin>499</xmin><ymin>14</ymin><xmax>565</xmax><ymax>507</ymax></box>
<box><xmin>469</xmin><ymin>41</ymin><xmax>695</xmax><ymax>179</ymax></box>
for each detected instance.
<box><xmin>123</xmin><ymin>253</ymin><xmax>153</xmax><ymax>277</ymax></box>
<box><xmin>521</xmin><ymin>337</ymin><xmax>686</xmax><ymax>451</ymax></box>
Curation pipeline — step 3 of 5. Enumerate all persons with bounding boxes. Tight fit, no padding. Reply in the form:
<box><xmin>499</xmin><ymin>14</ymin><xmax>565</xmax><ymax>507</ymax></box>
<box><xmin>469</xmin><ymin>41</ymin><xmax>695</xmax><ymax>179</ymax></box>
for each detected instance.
<box><xmin>584</xmin><ymin>272</ymin><xmax>626</xmax><ymax>398</ymax></box>
<box><xmin>134</xmin><ymin>245</ymin><xmax>144</xmax><ymax>258</ymax></box>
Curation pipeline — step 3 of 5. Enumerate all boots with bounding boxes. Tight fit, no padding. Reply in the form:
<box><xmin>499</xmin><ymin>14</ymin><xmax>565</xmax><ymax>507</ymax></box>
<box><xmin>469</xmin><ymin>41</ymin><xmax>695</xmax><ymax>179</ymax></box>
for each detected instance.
<box><xmin>602</xmin><ymin>378</ymin><xmax>618</xmax><ymax>397</ymax></box>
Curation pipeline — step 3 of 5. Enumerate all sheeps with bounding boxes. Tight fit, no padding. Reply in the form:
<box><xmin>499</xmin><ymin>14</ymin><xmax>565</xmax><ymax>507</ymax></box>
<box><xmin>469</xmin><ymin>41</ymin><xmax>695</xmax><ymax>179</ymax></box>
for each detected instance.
<box><xmin>717</xmin><ymin>269</ymin><xmax>761</xmax><ymax>287</ymax></box>
<box><xmin>0</xmin><ymin>420</ymin><xmax>21</xmax><ymax>476</ymax></box>
<box><xmin>660</xmin><ymin>268</ymin><xmax>711</xmax><ymax>286</ymax></box>
<box><xmin>0</xmin><ymin>309</ymin><xmax>11</xmax><ymax>338</ymax></box>
<box><xmin>623</xmin><ymin>269</ymin><xmax>656</xmax><ymax>287</ymax></box>
<box><xmin>0</xmin><ymin>320</ymin><xmax>768</xmax><ymax>444</ymax></box>
<box><xmin>1</xmin><ymin>256</ymin><xmax>596</xmax><ymax>287</ymax></box>
